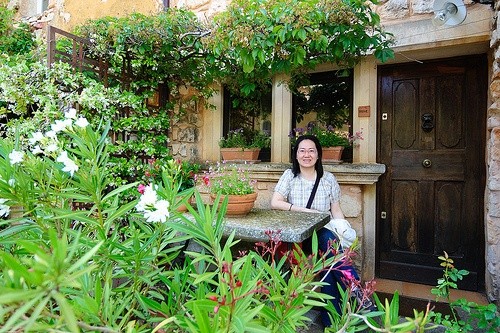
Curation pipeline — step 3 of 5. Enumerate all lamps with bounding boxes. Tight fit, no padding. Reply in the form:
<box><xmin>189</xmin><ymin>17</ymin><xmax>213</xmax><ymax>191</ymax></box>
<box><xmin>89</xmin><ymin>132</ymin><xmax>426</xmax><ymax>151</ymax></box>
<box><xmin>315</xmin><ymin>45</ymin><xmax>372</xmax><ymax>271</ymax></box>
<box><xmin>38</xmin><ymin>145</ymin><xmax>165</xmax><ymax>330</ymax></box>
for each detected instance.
<box><xmin>432</xmin><ymin>0</ymin><xmax>467</xmax><ymax>26</ymax></box>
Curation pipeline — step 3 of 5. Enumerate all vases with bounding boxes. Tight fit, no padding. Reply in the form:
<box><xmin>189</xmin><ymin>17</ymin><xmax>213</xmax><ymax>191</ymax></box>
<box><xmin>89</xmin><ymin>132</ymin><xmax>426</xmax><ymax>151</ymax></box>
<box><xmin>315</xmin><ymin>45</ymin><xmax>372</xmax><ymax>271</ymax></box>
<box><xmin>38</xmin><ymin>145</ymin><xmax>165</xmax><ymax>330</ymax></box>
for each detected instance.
<box><xmin>292</xmin><ymin>146</ymin><xmax>343</xmax><ymax>160</ymax></box>
<box><xmin>175</xmin><ymin>195</ymin><xmax>191</xmax><ymax>213</ymax></box>
<box><xmin>219</xmin><ymin>148</ymin><xmax>261</xmax><ymax>160</ymax></box>
<box><xmin>210</xmin><ymin>192</ymin><xmax>258</xmax><ymax>217</ymax></box>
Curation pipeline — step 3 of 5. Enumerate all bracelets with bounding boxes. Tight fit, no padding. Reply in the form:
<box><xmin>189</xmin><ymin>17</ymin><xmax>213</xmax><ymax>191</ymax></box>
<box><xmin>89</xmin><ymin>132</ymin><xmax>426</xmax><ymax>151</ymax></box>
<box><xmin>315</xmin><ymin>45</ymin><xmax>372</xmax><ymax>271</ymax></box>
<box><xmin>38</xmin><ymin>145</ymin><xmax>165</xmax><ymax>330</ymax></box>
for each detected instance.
<box><xmin>289</xmin><ymin>203</ymin><xmax>293</xmax><ymax>211</ymax></box>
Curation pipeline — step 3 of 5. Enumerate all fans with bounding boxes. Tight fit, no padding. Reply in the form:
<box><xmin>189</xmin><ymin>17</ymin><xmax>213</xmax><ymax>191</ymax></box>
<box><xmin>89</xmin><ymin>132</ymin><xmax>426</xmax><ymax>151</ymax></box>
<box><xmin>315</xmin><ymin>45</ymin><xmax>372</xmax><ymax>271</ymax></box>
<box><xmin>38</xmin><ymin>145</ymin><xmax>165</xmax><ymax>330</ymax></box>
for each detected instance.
<box><xmin>138</xmin><ymin>156</ymin><xmax>257</xmax><ymax>197</ymax></box>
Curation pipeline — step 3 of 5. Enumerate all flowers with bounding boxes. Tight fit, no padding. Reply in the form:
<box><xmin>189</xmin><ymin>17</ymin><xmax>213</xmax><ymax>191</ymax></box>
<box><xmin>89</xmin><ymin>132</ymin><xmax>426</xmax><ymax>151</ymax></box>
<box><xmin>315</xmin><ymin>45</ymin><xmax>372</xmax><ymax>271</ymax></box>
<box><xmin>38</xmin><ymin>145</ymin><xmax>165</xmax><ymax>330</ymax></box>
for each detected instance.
<box><xmin>288</xmin><ymin>123</ymin><xmax>363</xmax><ymax>155</ymax></box>
<box><xmin>218</xmin><ymin>128</ymin><xmax>271</xmax><ymax>152</ymax></box>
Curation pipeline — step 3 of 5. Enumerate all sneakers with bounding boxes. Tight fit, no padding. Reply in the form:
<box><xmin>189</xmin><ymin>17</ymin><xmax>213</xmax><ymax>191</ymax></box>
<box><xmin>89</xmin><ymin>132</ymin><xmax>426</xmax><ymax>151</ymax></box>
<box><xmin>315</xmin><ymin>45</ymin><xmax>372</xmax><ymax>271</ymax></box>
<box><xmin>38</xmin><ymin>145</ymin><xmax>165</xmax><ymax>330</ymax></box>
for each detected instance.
<box><xmin>349</xmin><ymin>290</ymin><xmax>378</xmax><ymax>315</ymax></box>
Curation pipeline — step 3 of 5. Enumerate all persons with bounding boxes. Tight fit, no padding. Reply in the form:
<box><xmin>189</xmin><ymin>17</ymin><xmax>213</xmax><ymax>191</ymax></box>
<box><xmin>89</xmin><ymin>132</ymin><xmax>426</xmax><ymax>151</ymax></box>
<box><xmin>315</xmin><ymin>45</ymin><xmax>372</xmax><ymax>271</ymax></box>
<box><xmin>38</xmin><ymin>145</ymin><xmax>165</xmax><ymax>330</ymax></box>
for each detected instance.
<box><xmin>271</xmin><ymin>135</ymin><xmax>378</xmax><ymax>327</ymax></box>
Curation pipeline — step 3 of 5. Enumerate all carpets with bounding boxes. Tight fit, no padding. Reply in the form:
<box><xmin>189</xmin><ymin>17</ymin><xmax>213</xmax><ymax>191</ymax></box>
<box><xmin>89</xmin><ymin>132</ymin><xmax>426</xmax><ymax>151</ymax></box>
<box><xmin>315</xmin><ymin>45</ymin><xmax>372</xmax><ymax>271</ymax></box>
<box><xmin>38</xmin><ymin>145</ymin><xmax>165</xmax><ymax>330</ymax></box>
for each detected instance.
<box><xmin>366</xmin><ymin>292</ymin><xmax>461</xmax><ymax>324</ymax></box>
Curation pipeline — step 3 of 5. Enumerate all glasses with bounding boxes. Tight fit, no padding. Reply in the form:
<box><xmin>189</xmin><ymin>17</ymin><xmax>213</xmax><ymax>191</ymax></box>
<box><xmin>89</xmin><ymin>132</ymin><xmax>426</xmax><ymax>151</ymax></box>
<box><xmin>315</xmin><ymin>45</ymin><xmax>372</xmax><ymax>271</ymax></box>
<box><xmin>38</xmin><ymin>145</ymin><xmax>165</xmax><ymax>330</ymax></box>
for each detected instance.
<box><xmin>298</xmin><ymin>149</ymin><xmax>317</xmax><ymax>154</ymax></box>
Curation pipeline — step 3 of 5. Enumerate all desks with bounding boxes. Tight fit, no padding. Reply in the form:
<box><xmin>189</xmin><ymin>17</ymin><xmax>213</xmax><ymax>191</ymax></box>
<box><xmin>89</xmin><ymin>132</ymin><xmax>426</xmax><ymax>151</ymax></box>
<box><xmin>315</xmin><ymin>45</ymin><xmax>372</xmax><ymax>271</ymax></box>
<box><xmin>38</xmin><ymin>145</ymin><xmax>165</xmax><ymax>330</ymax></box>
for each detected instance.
<box><xmin>132</xmin><ymin>209</ymin><xmax>330</xmax><ymax>282</ymax></box>
